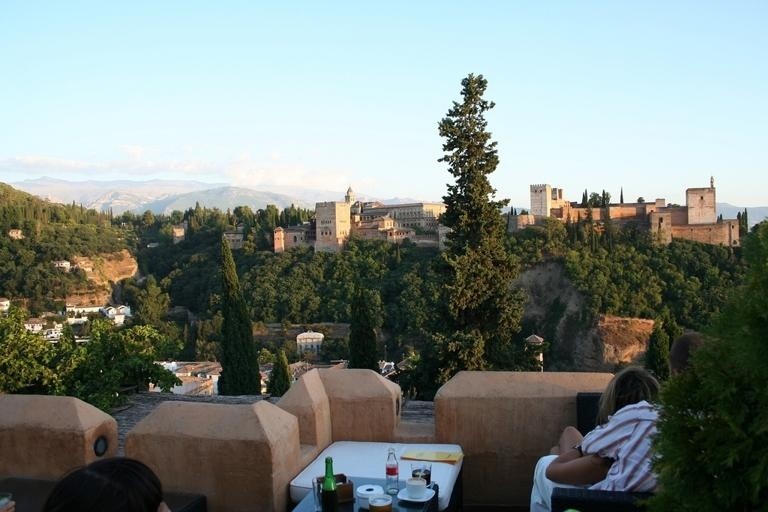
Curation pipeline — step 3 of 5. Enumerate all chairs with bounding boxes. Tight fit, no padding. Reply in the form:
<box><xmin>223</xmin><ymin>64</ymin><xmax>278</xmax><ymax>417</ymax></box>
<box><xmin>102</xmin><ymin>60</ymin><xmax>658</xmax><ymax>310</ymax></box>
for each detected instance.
<box><xmin>551</xmin><ymin>393</ymin><xmax>654</xmax><ymax>512</ymax></box>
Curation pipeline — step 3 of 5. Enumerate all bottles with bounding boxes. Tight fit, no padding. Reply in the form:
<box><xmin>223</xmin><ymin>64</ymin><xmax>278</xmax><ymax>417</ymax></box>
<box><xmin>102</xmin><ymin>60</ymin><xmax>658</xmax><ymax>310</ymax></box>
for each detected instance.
<box><xmin>385</xmin><ymin>448</ymin><xmax>399</xmax><ymax>495</ymax></box>
<box><xmin>320</xmin><ymin>456</ymin><xmax>337</xmax><ymax>511</ymax></box>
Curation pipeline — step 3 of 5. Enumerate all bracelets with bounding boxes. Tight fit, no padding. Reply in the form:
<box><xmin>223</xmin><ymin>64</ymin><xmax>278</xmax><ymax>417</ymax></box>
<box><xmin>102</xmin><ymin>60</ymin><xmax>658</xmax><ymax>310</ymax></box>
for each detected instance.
<box><xmin>571</xmin><ymin>444</ymin><xmax>583</xmax><ymax>456</ymax></box>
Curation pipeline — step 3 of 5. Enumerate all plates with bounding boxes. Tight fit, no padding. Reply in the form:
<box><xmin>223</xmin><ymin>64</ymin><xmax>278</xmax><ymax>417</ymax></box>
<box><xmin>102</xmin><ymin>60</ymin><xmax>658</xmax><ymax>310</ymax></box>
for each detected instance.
<box><xmin>397</xmin><ymin>487</ymin><xmax>435</xmax><ymax>503</ymax></box>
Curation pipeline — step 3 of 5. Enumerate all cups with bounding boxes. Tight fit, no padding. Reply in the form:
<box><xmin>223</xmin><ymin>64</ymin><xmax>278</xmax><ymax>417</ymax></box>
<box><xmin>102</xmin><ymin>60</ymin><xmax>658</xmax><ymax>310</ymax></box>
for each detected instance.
<box><xmin>406</xmin><ymin>460</ymin><xmax>432</xmax><ymax>498</ymax></box>
<box><xmin>368</xmin><ymin>493</ymin><xmax>392</xmax><ymax>512</ymax></box>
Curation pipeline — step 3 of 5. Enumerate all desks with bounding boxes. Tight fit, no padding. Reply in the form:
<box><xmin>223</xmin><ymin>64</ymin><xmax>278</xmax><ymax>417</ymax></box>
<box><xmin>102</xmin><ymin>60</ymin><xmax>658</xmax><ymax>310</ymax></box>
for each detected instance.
<box><xmin>292</xmin><ymin>476</ymin><xmax>439</xmax><ymax>512</ymax></box>
<box><xmin>290</xmin><ymin>441</ymin><xmax>464</xmax><ymax>510</ymax></box>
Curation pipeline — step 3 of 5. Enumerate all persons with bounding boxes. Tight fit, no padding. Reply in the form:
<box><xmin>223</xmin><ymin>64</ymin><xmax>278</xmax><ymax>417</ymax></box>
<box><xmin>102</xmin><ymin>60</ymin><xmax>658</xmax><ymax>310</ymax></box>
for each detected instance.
<box><xmin>527</xmin><ymin>333</ymin><xmax>709</xmax><ymax>511</ymax></box>
<box><xmin>0</xmin><ymin>500</ymin><xmax>16</xmax><ymax>511</ymax></box>
<box><xmin>41</xmin><ymin>455</ymin><xmax>172</xmax><ymax>511</ymax></box>
<box><xmin>544</xmin><ymin>365</ymin><xmax>665</xmax><ymax>484</ymax></box>
